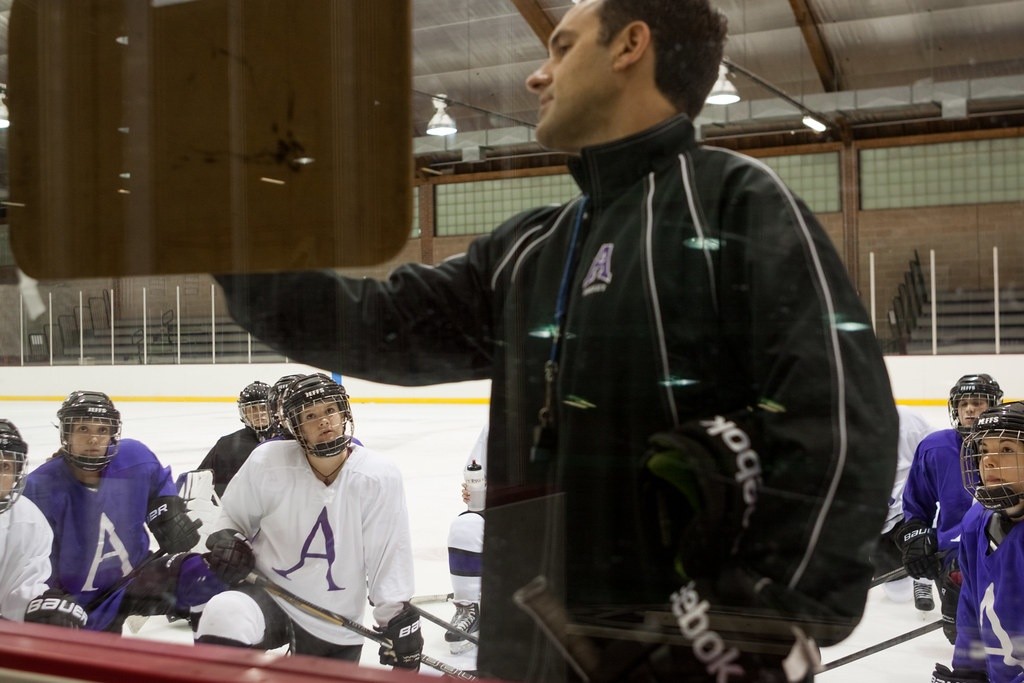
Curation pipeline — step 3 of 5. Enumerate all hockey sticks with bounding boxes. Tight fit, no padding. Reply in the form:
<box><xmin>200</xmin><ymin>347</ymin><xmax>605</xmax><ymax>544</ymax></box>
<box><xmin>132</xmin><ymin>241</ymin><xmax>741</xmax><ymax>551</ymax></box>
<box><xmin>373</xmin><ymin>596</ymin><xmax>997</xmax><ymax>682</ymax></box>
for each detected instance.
<box><xmin>82</xmin><ymin>518</ymin><xmax>204</xmax><ymax>615</ymax></box>
<box><xmin>242</xmin><ymin>573</ymin><xmax>480</xmax><ymax>683</ymax></box>
<box><xmin>803</xmin><ymin>564</ymin><xmax>947</xmax><ymax>676</ymax></box>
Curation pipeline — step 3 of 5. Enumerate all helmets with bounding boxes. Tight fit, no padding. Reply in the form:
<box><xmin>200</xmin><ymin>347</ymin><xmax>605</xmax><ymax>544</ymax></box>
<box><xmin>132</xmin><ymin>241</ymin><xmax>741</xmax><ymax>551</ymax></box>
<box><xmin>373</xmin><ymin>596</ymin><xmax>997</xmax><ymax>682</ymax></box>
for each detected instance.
<box><xmin>947</xmin><ymin>371</ymin><xmax>1002</xmax><ymax>434</ymax></box>
<box><xmin>55</xmin><ymin>389</ymin><xmax>123</xmax><ymax>473</ymax></box>
<box><xmin>238</xmin><ymin>380</ymin><xmax>280</xmax><ymax>434</ymax></box>
<box><xmin>267</xmin><ymin>373</ymin><xmax>308</xmax><ymax>439</ymax></box>
<box><xmin>0</xmin><ymin>418</ymin><xmax>29</xmax><ymax>514</ymax></box>
<box><xmin>959</xmin><ymin>401</ymin><xmax>1024</xmax><ymax>512</ymax></box>
<box><xmin>281</xmin><ymin>371</ymin><xmax>354</xmax><ymax>458</ymax></box>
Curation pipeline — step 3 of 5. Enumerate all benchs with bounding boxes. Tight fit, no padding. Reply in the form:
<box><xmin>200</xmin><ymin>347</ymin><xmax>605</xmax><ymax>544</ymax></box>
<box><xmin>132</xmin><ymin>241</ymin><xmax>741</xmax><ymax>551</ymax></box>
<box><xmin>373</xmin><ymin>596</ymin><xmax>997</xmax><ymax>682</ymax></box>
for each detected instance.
<box><xmin>905</xmin><ymin>291</ymin><xmax>1024</xmax><ymax>354</ymax></box>
<box><xmin>52</xmin><ymin>320</ymin><xmax>296</xmax><ymax>362</ymax></box>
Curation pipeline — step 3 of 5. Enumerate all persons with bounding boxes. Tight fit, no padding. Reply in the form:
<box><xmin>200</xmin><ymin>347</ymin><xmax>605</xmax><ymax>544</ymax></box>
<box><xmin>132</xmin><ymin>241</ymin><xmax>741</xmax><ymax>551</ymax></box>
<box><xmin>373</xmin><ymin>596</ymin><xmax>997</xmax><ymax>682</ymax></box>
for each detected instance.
<box><xmin>1</xmin><ymin>417</ymin><xmax>55</xmax><ymax>625</ymax></box>
<box><xmin>444</xmin><ymin>418</ymin><xmax>494</xmax><ymax>643</ymax></box>
<box><xmin>196</xmin><ymin>379</ymin><xmax>299</xmax><ymax>517</ymax></box>
<box><xmin>902</xmin><ymin>373</ymin><xmax>1002</xmax><ymax>644</ymax></box>
<box><xmin>208</xmin><ymin>1</ymin><xmax>904</xmax><ymax>683</ymax></box>
<box><xmin>195</xmin><ymin>371</ymin><xmax>424</xmax><ymax>670</ymax></box>
<box><xmin>19</xmin><ymin>391</ymin><xmax>215</xmax><ymax>636</ymax></box>
<box><xmin>929</xmin><ymin>398</ymin><xmax>1024</xmax><ymax>683</ymax></box>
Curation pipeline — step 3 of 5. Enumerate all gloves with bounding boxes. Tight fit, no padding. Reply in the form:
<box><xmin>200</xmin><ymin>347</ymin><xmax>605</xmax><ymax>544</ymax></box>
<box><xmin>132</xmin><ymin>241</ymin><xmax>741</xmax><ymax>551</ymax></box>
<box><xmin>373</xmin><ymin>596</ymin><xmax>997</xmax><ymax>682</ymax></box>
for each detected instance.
<box><xmin>893</xmin><ymin>517</ymin><xmax>941</xmax><ymax>581</ymax></box>
<box><xmin>145</xmin><ymin>495</ymin><xmax>201</xmax><ymax>556</ymax></box>
<box><xmin>21</xmin><ymin>588</ymin><xmax>89</xmax><ymax>631</ymax></box>
<box><xmin>382</xmin><ymin>606</ymin><xmax>424</xmax><ymax>674</ymax></box>
<box><xmin>202</xmin><ymin>528</ymin><xmax>255</xmax><ymax>587</ymax></box>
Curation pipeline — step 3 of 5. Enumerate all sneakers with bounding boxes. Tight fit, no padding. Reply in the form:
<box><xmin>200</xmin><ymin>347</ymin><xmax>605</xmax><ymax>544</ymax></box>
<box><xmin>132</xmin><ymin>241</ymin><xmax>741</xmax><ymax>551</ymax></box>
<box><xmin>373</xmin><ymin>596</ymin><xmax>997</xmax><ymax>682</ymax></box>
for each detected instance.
<box><xmin>443</xmin><ymin>600</ymin><xmax>480</xmax><ymax>657</ymax></box>
<box><xmin>913</xmin><ymin>580</ymin><xmax>936</xmax><ymax>611</ymax></box>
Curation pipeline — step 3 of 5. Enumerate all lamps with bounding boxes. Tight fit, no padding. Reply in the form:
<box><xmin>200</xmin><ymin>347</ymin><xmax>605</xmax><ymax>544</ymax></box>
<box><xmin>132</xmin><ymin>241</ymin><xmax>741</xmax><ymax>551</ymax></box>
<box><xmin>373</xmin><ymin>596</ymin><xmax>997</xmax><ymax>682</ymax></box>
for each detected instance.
<box><xmin>426</xmin><ymin>94</ymin><xmax>457</xmax><ymax>136</ymax></box>
<box><xmin>704</xmin><ymin>56</ymin><xmax>740</xmax><ymax>105</ymax></box>
